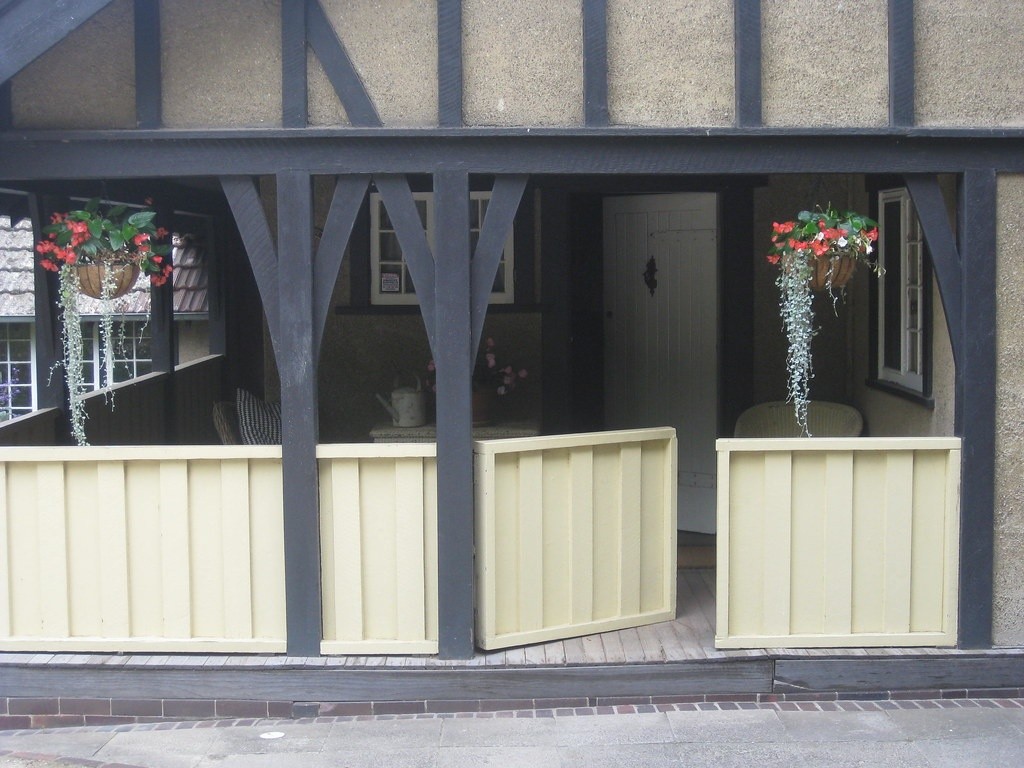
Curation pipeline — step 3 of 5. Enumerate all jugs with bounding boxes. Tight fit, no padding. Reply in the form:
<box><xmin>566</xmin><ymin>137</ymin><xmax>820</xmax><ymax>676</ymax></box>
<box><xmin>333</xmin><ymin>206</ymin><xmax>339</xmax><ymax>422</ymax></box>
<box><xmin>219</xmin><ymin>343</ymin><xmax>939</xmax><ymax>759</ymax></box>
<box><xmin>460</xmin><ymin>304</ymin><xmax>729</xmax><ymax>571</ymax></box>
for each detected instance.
<box><xmin>375</xmin><ymin>371</ymin><xmax>427</xmax><ymax>427</ymax></box>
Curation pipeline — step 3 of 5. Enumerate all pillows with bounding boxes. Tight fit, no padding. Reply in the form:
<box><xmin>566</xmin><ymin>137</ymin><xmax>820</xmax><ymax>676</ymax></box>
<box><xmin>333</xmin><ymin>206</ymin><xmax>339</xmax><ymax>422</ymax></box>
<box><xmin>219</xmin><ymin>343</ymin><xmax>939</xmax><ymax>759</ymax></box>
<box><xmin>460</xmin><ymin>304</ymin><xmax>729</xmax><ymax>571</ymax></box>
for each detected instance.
<box><xmin>237</xmin><ymin>388</ymin><xmax>281</xmax><ymax>445</ymax></box>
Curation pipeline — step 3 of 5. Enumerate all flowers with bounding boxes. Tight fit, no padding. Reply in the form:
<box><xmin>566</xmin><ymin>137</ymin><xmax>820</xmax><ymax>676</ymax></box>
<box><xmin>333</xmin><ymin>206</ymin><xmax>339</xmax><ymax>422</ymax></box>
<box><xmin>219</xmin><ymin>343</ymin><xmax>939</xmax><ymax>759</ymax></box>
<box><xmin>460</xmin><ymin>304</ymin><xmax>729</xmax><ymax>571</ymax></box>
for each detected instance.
<box><xmin>36</xmin><ymin>187</ymin><xmax>173</xmax><ymax>446</ymax></box>
<box><xmin>766</xmin><ymin>200</ymin><xmax>885</xmax><ymax>438</ymax></box>
<box><xmin>426</xmin><ymin>338</ymin><xmax>529</xmax><ymax>394</ymax></box>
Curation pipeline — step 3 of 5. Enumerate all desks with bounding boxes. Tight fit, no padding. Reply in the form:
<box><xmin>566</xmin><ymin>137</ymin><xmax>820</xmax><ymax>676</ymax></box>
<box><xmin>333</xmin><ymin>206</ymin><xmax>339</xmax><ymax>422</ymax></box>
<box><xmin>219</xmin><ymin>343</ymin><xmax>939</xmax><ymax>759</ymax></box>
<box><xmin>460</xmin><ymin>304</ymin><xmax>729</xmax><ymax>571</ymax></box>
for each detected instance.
<box><xmin>370</xmin><ymin>387</ymin><xmax>542</xmax><ymax>443</ymax></box>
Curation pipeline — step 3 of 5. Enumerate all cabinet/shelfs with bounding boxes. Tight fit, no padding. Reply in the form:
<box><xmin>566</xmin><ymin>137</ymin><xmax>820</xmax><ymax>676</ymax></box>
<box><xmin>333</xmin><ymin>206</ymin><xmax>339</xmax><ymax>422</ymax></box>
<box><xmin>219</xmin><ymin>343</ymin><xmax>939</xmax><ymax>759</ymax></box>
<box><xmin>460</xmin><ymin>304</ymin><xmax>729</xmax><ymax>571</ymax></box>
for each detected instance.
<box><xmin>370</xmin><ymin>190</ymin><xmax>515</xmax><ymax>304</ymax></box>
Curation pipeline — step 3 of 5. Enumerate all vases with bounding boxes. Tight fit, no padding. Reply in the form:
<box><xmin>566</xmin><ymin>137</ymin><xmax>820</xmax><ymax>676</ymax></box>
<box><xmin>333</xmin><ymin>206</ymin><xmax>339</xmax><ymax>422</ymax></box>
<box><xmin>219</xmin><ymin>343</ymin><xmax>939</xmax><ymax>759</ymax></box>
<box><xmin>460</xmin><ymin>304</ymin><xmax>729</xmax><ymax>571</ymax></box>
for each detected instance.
<box><xmin>785</xmin><ymin>253</ymin><xmax>857</xmax><ymax>290</ymax></box>
<box><xmin>72</xmin><ymin>249</ymin><xmax>140</xmax><ymax>300</ymax></box>
<box><xmin>457</xmin><ymin>376</ymin><xmax>495</xmax><ymax>427</ymax></box>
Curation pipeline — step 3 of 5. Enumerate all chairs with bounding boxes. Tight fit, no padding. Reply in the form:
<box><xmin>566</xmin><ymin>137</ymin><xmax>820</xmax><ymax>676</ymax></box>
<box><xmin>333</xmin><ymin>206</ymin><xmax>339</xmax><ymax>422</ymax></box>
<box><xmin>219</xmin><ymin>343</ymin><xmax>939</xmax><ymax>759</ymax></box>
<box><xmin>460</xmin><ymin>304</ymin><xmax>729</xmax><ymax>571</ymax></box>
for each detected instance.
<box><xmin>212</xmin><ymin>400</ymin><xmax>243</xmax><ymax>446</ymax></box>
<box><xmin>733</xmin><ymin>400</ymin><xmax>863</xmax><ymax>438</ymax></box>
